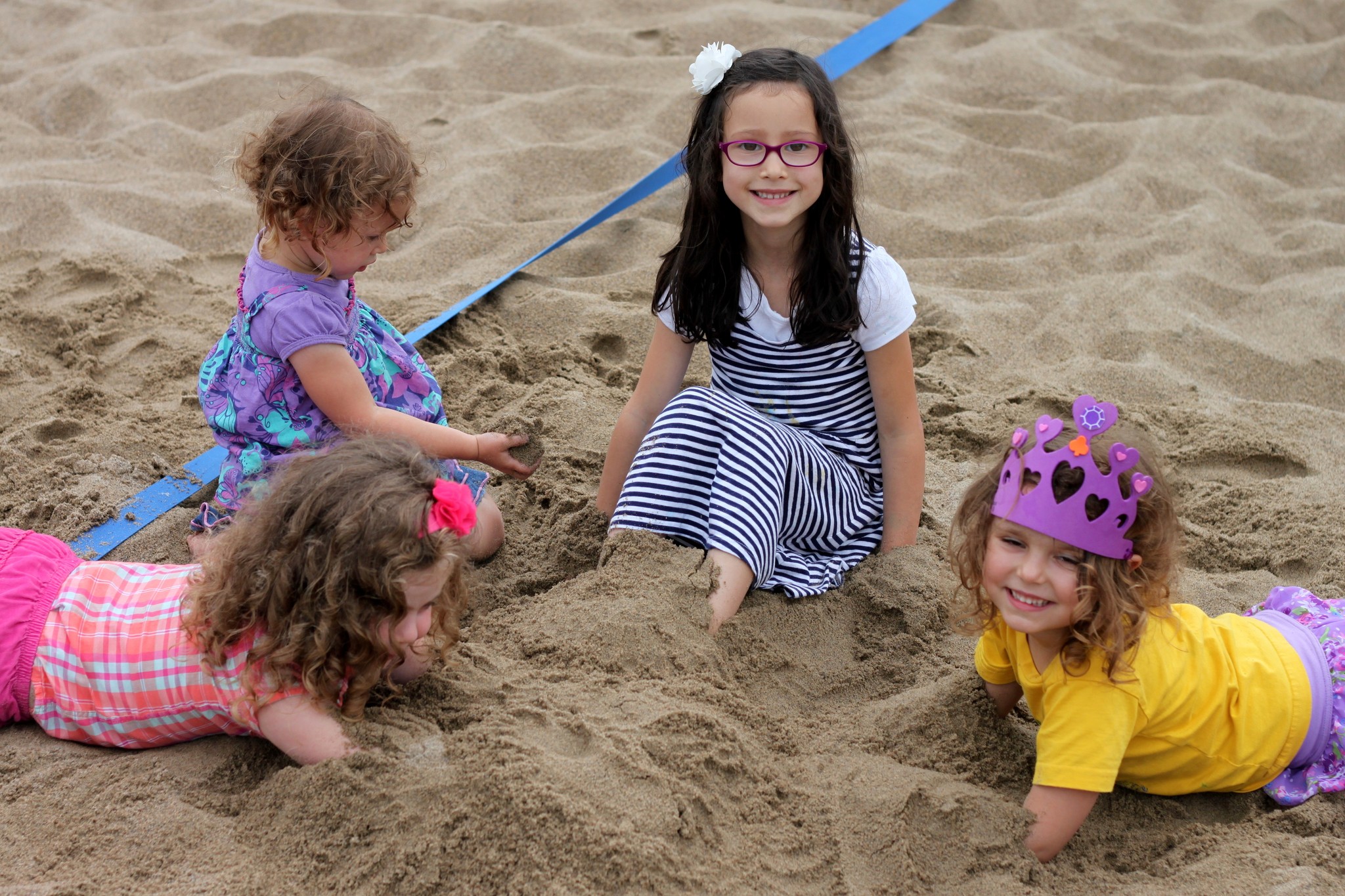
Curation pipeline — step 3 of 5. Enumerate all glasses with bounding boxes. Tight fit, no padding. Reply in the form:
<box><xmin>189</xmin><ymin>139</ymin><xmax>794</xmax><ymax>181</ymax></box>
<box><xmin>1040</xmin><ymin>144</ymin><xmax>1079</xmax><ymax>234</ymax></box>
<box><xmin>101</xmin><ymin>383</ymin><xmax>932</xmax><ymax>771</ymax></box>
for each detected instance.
<box><xmin>716</xmin><ymin>139</ymin><xmax>827</xmax><ymax>167</ymax></box>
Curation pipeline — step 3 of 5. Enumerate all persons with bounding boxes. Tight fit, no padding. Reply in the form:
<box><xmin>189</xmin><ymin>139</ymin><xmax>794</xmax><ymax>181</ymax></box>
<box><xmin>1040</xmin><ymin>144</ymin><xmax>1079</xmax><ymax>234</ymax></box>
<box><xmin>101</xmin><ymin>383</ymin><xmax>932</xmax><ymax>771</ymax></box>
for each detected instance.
<box><xmin>595</xmin><ymin>42</ymin><xmax>927</xmax><ymax>631</ymax></box>
<box><xmin>946</xmin><ymin>392</ymin><xmax>1345</xmax><ymax>864</ymax></box>
<box><xmin>1</xmin><ymin>423</ymin><xmax>477</xmax><ymax>768</ymax></box>
<box><xmin>199</xmin><ymin>91</ymin><xmax>543</xmax><ymax>560</ymax></box>
